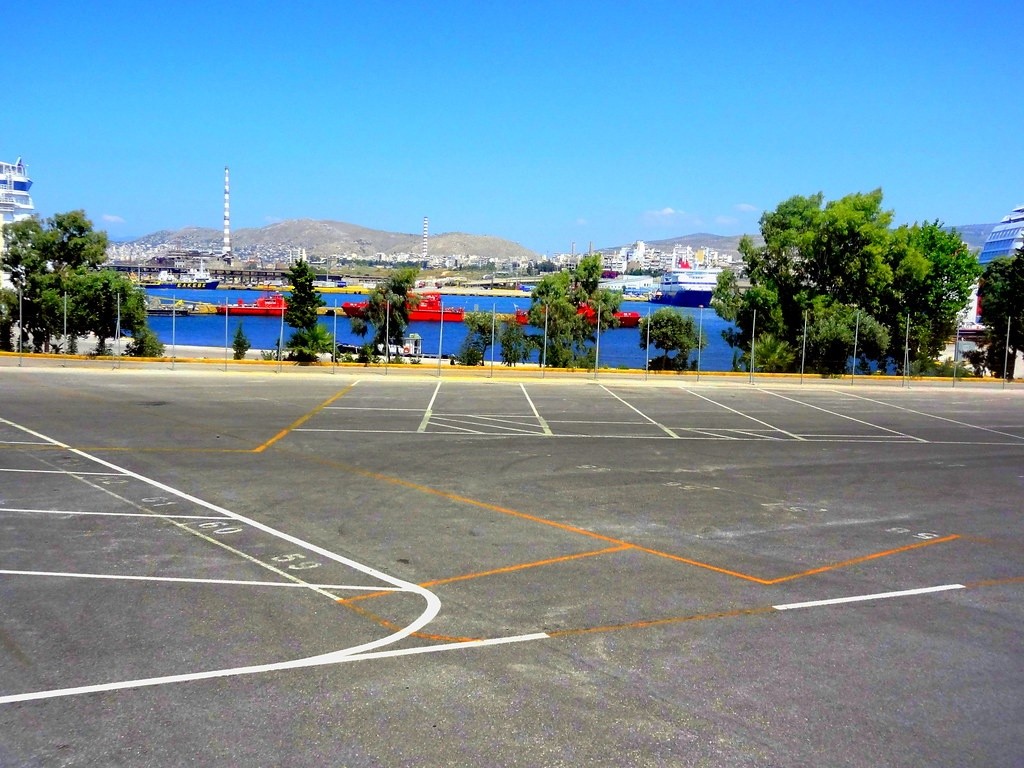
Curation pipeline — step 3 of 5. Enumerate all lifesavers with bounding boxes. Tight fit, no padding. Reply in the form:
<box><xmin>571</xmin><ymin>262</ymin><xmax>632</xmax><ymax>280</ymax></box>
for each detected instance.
<box><xmin>404</xmin><ymin>347</ymin><xmax>409</xmax><ymax>353</ymax></box>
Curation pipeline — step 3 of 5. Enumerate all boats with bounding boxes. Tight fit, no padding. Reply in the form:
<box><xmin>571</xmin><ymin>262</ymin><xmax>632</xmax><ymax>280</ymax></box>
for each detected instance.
<box><xmin>341</xmin><ymin>292</ymin><xmax>464</xmax><ymax>321</ymax></box>
<box><xmin>215</xmin><ymin>291</ymin><xmax>289</xmax><ymax>317</ymax></box>
<box><xmin>647</xmin><ymin>262</ymin><xmax>723</xmax><ymax>308</ymax></box>
<box><xmin>337</xmin><ymin>333</ymin><xmax>438</xmax><ymax>358</ymax></box>
<box><xmin>143</xmin><ymin>296</ymin><xmax>189</xmax><ymax>317</ymax></box>
<box><xmin>516</xmin><ymin>298</ymin><xmax>641</xmax><ymax>328</ymax></box>
<box><xmin>144</xmin><ymin>259</ymin><xmax>222</xmax><ymax>290</ymax></box>
